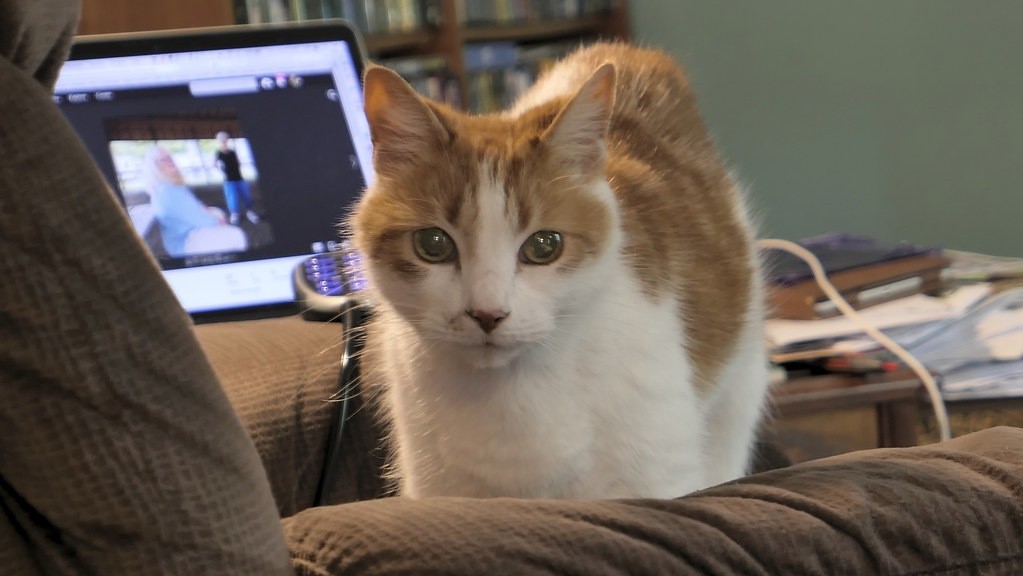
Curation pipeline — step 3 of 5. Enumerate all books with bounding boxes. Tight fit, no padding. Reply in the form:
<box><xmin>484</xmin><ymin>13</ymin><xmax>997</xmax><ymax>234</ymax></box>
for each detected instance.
<box><xmin>243</xmin><ymin>0</ymin><xmax>616</xmax><ymax>115</ymax></box>
<box><xmin>749</xmin><ymin>228</ymin><xmax>951</xmax><ymax>322</ymax></box>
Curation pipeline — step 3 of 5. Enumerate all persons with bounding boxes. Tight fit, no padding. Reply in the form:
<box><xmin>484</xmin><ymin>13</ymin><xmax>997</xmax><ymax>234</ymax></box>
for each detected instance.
<box><xmin>212</xmin><ymin>131</ymin><xmax>260</xmax><ymax>225</ymax></box>
<box><xmin>149</xmin><ymin>155</ymin><xmax>225</xmax><ymax>258</ymax></box>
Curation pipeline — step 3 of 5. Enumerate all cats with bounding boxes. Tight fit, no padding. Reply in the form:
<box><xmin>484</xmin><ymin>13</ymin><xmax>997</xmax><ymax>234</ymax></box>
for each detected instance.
<box><xmin>315</xmin><ymin>39</ymin><xmax>782</xmax><ymax>500</ymax></box>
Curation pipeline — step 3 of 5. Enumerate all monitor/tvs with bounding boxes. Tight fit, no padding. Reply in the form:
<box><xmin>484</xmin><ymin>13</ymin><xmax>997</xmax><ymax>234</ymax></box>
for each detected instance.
<box><xmin>53</xmin><ymin>21</ymin><xmax>379</xmax><ymax>332</ymax></box>
